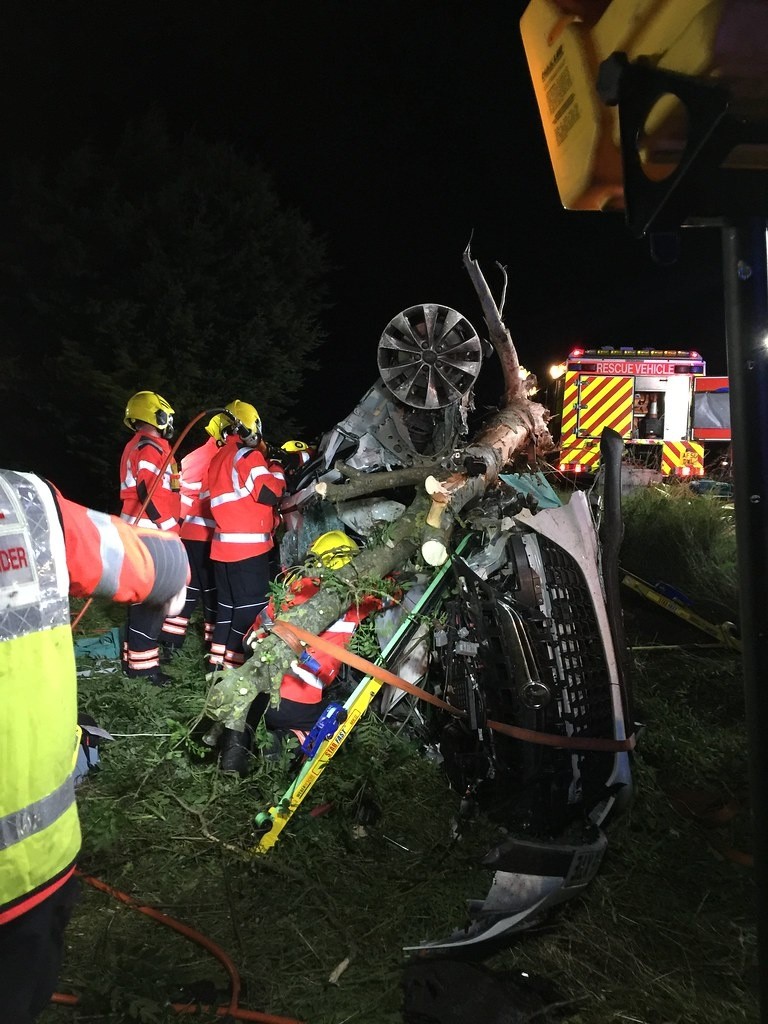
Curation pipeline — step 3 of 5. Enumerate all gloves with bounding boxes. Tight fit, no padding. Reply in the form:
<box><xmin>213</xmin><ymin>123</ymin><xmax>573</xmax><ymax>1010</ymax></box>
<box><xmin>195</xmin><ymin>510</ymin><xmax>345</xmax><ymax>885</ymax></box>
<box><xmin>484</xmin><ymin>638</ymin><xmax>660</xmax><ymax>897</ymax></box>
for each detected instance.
<box><xmin>269</xmin><ymin>447</ymin><xmax>289</xmax><ymax>468</ymax></box>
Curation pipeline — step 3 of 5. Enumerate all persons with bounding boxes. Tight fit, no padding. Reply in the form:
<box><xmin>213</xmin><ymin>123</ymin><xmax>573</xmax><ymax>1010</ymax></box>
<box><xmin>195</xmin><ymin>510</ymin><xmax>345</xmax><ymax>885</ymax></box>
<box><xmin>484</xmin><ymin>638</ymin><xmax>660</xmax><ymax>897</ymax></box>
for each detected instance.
<box><xmin>119</xmin><ymin>391</ymin><xmax>181</xmax><ymax>686</ymax></box>
<box><xmin>0</xmin><ymin>469</ymin><xmax>189</xmax><ymax>1023</ymax></box>
<box><xmin>177</xmin><ymin>414</ymin><xmax>221</xmax><ymax>526</ymax></box>
<box><xmin>191</xmin><ymin>530</ymin><xmax>403</xmax><ymax>778</ymax></box>
<box><xmin>204</xmin><ymin>399</ymin><xmax>286</xmax><ymax>688</ymax></box>
<box><xmin>159</xmin><ymin>470</ymin><xmax>217</xmax><ymax>665</ymax></box>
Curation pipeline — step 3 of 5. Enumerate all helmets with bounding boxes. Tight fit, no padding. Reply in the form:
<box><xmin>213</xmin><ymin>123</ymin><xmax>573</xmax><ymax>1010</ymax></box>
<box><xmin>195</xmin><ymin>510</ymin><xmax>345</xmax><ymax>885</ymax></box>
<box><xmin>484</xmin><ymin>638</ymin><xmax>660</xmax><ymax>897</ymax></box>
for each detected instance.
<box><xmin>123</xmin><ymin>391</ymin><xmax>175</xmax><ymax>440</ymax></box>
<box><xmin>219</xmin><ymin>399</ymin><xmax>263</xmax><ymax>437</ymax></box>
<box><xmin>281</xmin><ymin>440</ymin><xmax>309</xmax><ymax>454</ymax></box>
<box><xmin>308</xmin><ymin>530</ymin><xmax>361</xmax><ymax>570</ymax></box>
<box><xmin>205</xmin><ymin>412</ymin><xmax>223</xmax><ymax>450</ymax></box>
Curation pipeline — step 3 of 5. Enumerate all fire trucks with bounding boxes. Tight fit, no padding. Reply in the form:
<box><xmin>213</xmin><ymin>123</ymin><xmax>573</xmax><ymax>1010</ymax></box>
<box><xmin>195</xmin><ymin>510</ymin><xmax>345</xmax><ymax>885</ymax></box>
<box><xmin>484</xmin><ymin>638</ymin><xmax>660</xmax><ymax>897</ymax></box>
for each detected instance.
<box><xmin>545</xmin><ymin>345</ymin><xmax>731</xmax><ymax>488</ymax></box>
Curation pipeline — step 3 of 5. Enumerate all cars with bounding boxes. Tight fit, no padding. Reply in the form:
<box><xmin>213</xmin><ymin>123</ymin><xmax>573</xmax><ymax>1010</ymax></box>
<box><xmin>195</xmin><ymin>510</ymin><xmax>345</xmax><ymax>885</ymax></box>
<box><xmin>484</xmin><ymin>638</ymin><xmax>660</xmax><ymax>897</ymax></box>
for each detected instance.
<box><xmin>271</xmin><ymin>304</ymin><xmax>641</xmax><ymax>966</ymax></box>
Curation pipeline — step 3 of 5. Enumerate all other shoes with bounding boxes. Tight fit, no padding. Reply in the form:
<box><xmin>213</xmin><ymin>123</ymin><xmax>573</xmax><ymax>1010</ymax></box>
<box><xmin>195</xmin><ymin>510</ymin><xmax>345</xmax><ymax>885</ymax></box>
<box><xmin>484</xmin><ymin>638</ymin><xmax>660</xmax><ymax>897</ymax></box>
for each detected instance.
<box><xmin>189</xmin><ymin>716</ymin><xmax>259</xmax><ymax>780</ymax></box>
<box><xmin>120</xmin><ymin>665</ymin><xmax>178</xmax><ymax>689</ymax></box>
